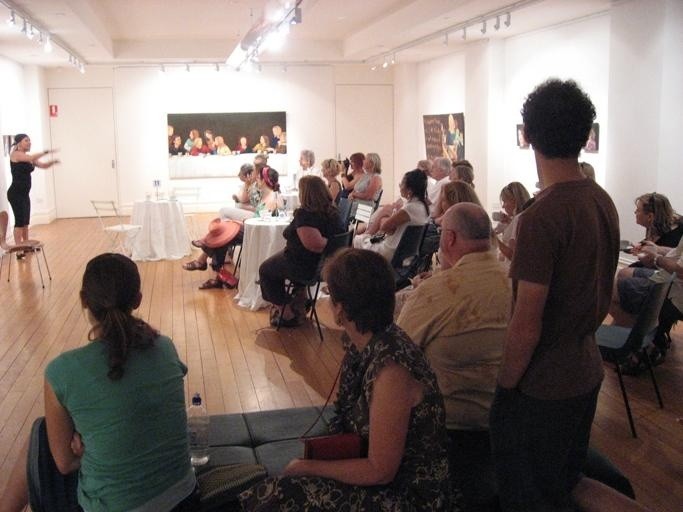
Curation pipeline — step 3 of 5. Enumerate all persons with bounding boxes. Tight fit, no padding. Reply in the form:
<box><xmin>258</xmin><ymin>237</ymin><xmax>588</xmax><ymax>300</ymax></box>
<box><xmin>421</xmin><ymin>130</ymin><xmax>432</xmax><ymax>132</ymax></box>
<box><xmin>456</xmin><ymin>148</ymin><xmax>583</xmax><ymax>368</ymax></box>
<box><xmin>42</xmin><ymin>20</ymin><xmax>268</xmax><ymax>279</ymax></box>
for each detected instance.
<box><xmin>6</xmin><ymin>135</ymin><xmax>61</xmax><ymax>257</ymax></box>
<box><xmin>490</xmin><ymin>79</ymin><xmax>620</xmax><ymax>511</ymax></box>
<box><xmin>182</xmin><ymin>156</ymin><xmax>284</xmax><ymax>290</ymax></box>
<box><xmin>517</xmin><ymin>128</ymin><xmax>528</xmax><ymax>147</ymax></box>
<box><xmin>44</xmin><ymin>255</ymin><xmax>199</xmax><ymax>510</ymax></box>
<box><xmin>610</xmin><ymin>182</ymin><xmax>683</xmax><ymax>376</ymax></box>
<box><xmin>295</xmin><ymin>150</ymin><xmax>382</xmax><ymax>207</ymax></box>
<box><xmin>262</xmin><ymin>175</ymin><xmax>344</xmax><ymax>332</ymax></box>
<box><xmin>435</xmin><ymin>115</ymin><xmax>463</xmax><ymax>164</ymax></box>
<box><xmin>167</xmin><ymin>121</ymin><xmax>287</xmax><ymax>156</ymax></box>
<box><xmin>242</xmin><ymin>245</ymin><xmax>453</xmax><ymax>509</ymax></box>
<box><xmin>576</xmin><ymin>162</ymin><xmax>596</xmax><ymax>185</ymax></box>
<box><xmin>392</xmin><ymin>203</ymin><xmax>519</xmax><ymax>462</ymax></box>
<box><xmin>356</xmin><ymin>157</ymin><xmax>532</xmax><ymax>285</ymax></box>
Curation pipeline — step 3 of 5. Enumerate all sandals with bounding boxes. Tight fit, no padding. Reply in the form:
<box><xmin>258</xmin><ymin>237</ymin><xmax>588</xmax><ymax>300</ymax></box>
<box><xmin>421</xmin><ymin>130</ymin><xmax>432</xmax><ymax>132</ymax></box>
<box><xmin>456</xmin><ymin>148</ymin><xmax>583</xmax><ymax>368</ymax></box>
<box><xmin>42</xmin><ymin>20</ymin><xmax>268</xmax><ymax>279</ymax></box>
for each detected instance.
<box><xmin>183</xmin><ymin>260</ymin><xmax>207</xmax><ymax>270</ymax></box>
<box><xmin>198</xmin><ymin>279</ymin><xmax>223</xmax><ymax>289</ymax></box>
<box><xmin>621</xmin><ymin>346</ymin><xmax>665</xmax><ymax>375</ymax></box>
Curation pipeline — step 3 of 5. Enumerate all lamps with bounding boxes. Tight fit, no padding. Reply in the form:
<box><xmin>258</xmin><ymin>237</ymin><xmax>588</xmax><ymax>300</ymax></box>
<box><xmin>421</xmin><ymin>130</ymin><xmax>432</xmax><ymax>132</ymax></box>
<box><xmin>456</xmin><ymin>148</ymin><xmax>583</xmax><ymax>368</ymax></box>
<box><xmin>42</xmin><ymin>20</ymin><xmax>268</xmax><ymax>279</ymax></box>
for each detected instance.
<box><xmin>160</xmin><ymin>61</ymin><xmax>289</xmax><ymax>74</ymax></box>
<box><xmin>362</xmin><ymin>0</ymin><xmax>541</xmax><ymax>71</ymax></box>
<box><xmin>288</xmin><ymin>7</ymin><xmax>302</xmax><ymax>24</ymax></box>
<box><xmin>0</xmin><ymin>0</ymin><xmax>87</xmax><ymax>75</ymax></box>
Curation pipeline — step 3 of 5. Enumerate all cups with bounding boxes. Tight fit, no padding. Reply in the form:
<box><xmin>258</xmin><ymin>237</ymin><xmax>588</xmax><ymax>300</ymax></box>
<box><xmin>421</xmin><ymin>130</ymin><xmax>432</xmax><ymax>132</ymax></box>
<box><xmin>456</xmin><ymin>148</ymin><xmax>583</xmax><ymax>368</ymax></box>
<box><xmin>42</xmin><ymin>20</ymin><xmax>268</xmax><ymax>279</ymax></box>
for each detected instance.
<box><xmin>259</xmin><ymin>208</ymin><xmax>294</xmax><ymax>223</ymax></box>
<box><xmin>169</xmin><ymin>150</ymin><xmax>267</xmax><ymax>157</ymax></box>
<box><xmin>145</xmin><ymin>191</ymin><xmax>178</xmax><ymax>201</ymax></box>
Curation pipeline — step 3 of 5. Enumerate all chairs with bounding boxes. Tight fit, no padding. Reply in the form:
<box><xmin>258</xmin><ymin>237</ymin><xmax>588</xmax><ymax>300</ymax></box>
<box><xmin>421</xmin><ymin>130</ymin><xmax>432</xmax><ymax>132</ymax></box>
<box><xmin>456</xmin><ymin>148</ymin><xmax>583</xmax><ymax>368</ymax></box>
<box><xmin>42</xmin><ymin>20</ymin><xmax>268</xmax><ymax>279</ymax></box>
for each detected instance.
<box><xmin>0</xmin><ymin>211</ymin><xmax>51</xmax><ymax>289</ymax></box>
<box><xmin>91</xmin><ymin>200</ymin><xmax>145</xmax><ymax>262</ymax></box>
<box><xmin>231</xmin><ymin>199</ymin><xmax>287</xmax><ymax>276</ymax></box>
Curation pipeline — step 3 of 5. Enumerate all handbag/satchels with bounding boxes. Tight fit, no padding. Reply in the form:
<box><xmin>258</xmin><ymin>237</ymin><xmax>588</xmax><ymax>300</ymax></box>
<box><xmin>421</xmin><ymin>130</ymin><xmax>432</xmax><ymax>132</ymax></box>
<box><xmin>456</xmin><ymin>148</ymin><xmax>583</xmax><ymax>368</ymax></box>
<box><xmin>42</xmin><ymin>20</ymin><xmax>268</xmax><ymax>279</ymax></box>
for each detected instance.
<box><xmin>370</xmin><ymin>232</ymin><xmax>386</xmax><ymax>243</ymax></box>
<box><xmin>218</xmin><ymin>268</ymin><xmax>239</xmax><ymax>288</ymax></box>
<box><xmin>304</xmin><ymin>434</ymin><xmax>361</xmax><ymax>460</ymax></box>
<box><xmin>269</xmin><ymin>296</ymin><xmax>306</xmax><ymax>324</ymax></box>
<box><xmin>196</xmin><ymin>464</ymin><xmax>268</xmax><ymax>508</ymax></box>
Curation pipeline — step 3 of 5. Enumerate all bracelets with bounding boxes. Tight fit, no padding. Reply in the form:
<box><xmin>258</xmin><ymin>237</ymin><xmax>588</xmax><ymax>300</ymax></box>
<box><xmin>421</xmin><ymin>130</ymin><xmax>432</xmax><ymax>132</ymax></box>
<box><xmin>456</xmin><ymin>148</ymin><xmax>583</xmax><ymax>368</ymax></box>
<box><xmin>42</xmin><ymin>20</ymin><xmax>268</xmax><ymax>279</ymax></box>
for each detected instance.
<box><xmin>44</xmin><ymin>150</ymin><xmax>49</xmax><ymax>154</ymax></box>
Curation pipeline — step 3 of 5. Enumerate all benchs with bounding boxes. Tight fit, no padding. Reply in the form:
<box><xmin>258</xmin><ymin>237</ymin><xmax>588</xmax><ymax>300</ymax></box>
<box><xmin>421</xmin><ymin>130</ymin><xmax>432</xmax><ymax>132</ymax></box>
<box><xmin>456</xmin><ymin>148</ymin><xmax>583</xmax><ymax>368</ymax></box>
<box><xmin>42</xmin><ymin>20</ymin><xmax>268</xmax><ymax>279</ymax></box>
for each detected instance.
<box><xmin>27</xmin><ymin>405</ymin><xmax>636</xmax><ymax>511</ymax></box>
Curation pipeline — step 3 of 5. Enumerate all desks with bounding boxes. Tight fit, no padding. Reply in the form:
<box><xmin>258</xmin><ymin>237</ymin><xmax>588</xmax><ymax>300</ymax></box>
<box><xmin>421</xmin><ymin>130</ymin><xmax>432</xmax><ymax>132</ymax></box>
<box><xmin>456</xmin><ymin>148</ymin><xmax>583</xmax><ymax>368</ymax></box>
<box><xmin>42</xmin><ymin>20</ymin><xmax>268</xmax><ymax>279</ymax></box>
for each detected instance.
<box><xmin>125</xmin><ymin>200</ymin><xmax>192</xmax><ymax>261</ymax></box>
<box><xmin>233</xmin><ymin>217</ymin><xmax>330</xmax><ymax>312</ymax></box>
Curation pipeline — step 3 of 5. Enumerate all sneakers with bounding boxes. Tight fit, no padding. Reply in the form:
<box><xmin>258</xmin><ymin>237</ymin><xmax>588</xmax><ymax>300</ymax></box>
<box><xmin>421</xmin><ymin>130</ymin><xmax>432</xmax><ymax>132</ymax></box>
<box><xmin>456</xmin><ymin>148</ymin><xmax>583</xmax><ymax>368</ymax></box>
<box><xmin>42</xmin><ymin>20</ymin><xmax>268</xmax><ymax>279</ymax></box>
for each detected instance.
<box><xmin>192</xmin><ymin>240</ymin><xmax>205</xmax><ymax>247</ymax></box>
<box><xmin>17</xmin><ymin>248</ymin><xmax>40</xmax><ymax>259</ymax></box>
<box><xmin>271</xmin><ymin>314</ymin><xmax>299</xmax><ymax>326</ymax></box>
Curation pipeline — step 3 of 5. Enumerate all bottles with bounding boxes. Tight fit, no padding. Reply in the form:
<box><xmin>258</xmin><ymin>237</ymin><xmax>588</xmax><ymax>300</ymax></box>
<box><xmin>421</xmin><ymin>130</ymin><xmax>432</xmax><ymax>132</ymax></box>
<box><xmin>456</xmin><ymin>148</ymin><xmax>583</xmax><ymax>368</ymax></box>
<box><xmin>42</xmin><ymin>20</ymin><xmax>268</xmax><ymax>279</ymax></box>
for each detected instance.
<box><xmin>187</xmin><ymin>392</ymin><xmax>210</xmax><ymax>466</ymax></box>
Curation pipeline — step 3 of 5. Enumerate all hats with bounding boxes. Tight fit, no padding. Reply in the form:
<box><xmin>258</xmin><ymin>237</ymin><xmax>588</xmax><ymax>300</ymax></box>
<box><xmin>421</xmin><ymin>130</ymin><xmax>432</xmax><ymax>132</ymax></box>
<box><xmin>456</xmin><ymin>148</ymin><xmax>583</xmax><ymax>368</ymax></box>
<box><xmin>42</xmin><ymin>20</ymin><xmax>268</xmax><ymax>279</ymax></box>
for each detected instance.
<box><xmin>11</xmin><ymin>134</ymin><xmax>26</xmax><ymax>146</ymax></box>
<box><xmin>203</xmin><ymin>218</ymin><xmax>241</xmax><ymax>249</ymax></box>
